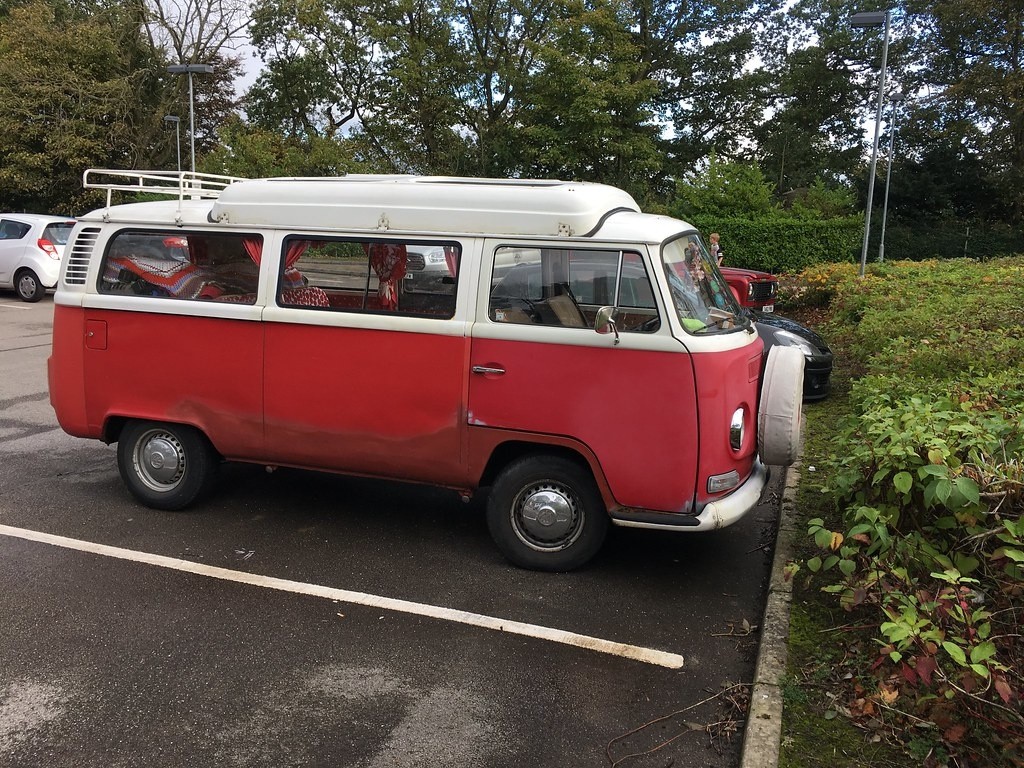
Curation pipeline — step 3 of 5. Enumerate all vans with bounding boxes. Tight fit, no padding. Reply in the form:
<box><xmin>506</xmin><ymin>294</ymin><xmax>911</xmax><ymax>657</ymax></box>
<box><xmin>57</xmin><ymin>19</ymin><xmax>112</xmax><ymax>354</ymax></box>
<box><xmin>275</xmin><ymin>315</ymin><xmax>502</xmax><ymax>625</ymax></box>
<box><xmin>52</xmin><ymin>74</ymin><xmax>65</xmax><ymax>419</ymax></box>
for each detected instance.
<box><xmin>48</xmin><ymin>167</ymin><xmax>807</xmax><ymax>571</ymax></box>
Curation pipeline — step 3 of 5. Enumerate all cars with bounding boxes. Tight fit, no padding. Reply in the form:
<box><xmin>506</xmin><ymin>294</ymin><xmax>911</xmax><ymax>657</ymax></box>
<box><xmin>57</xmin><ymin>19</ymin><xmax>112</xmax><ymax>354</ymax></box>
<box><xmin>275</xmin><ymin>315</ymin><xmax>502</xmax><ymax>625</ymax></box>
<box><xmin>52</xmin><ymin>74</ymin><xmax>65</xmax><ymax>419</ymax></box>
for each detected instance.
<box><xmin>0</xmin><ymin>214</ymin><xmax>77</xmax><ymax>303</ymax></box>
<box><xmin>406</xmin><ymin>240</ymin><xmax>833</xmax><ymax>404</ymax></box>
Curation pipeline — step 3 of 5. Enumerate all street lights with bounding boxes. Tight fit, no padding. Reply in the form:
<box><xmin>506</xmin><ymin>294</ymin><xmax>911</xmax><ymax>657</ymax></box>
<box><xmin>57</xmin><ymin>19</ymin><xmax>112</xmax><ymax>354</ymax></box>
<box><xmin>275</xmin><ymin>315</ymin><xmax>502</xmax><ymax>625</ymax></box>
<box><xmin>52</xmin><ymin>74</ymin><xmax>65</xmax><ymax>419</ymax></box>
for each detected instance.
<box><xmin>877</xmin><ymin>93</ymin><xmax>904</xmax><ymax>264</ymax></box>
<box><xmin>163</xmin><ymin>116</ymin><xmax>181</xmax><ymax>172</ymax></box>
<box><xmin>849</xmin><ymin>11</ymin><xmax>891</xmax><ymax>276</ymax></box>
<box><xmin>167</xmin><ymin>65</ymin><xmax>214</xmax><ymax>180</ymax></box>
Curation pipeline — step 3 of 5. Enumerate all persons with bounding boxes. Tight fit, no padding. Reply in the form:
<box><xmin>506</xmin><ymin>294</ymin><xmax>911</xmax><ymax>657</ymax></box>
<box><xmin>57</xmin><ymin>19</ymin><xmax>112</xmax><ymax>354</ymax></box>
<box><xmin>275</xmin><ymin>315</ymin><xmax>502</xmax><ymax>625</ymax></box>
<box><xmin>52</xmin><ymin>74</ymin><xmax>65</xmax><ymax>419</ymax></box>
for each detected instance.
<box><xmin>710</xmin><ymin>233</ymin><xmax>723</xmax><ymax>267</ymax></box>
<box><xmin>685</xmin><ymin>243</ymin><xmax>704</xmax><ymax>289</ymax></box>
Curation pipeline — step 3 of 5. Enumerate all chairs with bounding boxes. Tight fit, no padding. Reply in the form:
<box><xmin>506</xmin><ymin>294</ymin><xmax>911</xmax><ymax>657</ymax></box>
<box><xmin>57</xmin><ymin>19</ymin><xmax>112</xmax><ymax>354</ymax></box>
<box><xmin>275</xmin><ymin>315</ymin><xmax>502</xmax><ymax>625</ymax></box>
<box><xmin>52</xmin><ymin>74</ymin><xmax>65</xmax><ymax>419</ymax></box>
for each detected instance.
<box><xmin>214</xmin><ymin>287</ymin><xmax>330</xmax><ymax>309</ymax></box>
<box><xmin>492</xmin><ymin>294</ymin><xmax>586</xmax><ymax>330</ymax></box>
